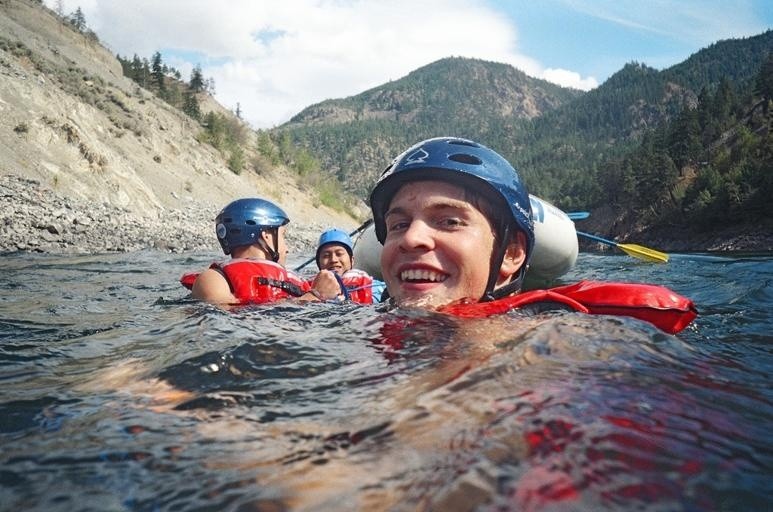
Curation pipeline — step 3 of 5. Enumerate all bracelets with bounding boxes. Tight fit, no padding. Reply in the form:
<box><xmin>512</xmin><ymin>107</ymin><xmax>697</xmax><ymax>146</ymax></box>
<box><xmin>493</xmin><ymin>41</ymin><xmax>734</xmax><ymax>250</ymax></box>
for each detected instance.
<box><xmin>305</xmin><ymin>286</ymin><xmax>327</xmax><ymax>303</ymax></box>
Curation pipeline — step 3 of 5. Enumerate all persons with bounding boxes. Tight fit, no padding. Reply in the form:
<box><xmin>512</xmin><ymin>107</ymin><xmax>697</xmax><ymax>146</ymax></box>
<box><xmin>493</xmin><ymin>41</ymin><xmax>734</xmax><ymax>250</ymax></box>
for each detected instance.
<box><xmin>71</xmin><ymin>136</ymin><xmax>728</xmax><ymax>511</ymax></box>
<box><xmin>177</xmin><ymin>197</ymin><xmax>344</xmax><ymax>312</ymax></box>
<box><xmin>305</xmin><ymin>228</ymin><xmax>386</xmax><ymax>304</ymax></box>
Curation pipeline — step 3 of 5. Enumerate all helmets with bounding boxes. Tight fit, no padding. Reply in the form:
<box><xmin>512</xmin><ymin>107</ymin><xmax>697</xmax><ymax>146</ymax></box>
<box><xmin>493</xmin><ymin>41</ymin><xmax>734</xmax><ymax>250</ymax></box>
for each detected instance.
<box><xmin>366</xmin><ymin>136</ymin><xmax>538</xmax><ymax>282</ymax></box>
<box><xmin>213</xmin><ymin>196</ymin><xmax>289</xmax><ymax>256</ymax></box>
<box><xmin>315</xmin><ymin>229</ymin><xmax>356</xmax><ymax>264</ymax></box>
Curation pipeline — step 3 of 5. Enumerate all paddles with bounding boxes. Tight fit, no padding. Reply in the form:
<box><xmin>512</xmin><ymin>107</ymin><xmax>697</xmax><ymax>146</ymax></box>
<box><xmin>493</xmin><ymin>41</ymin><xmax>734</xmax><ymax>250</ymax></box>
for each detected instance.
<box><xmin>567</xmin><ymin>213</ymin><xmax>590</xmax><ymax>220</ymax></box>
<box><xmin>576</xmin><ymin>231</ymin><xmax>668</xmax><ymax>264</ymax></box>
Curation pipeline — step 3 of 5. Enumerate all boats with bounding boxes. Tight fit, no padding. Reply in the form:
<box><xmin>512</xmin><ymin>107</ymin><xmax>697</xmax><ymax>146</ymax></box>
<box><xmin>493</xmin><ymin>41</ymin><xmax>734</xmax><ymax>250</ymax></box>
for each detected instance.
<box><xmin>351</xmin><ymin>188</ymin><xmax>578</xmax><ymax>290</ymax></box>
